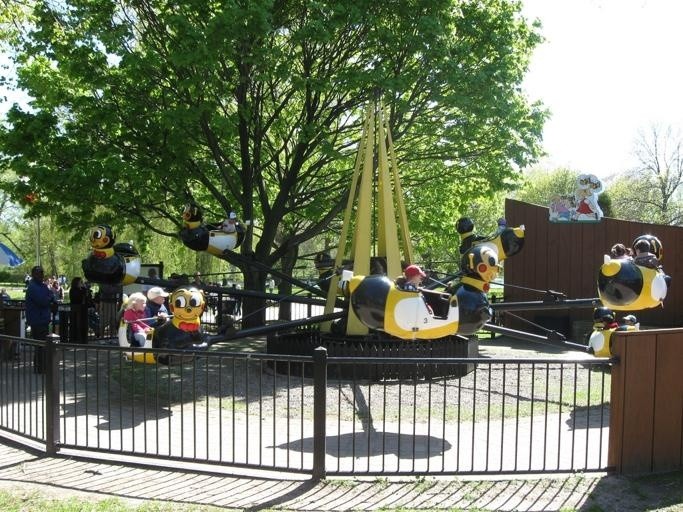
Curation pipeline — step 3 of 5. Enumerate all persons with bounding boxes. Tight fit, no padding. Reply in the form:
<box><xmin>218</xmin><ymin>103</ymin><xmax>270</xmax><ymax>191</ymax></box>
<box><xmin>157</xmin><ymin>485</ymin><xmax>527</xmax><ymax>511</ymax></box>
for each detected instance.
<box><xmin>403</xmin><ymin>265</ymin><xmax>435</xmax><ymax>318</ymax></box>
<box><xmin>611</xmin><ymin>243</ymin><xmax>629</xmax><ymax>258</ymax></box>
<box><xmin>622</xmin><ymin>314</ymin><xmax>637</xmax><ymax>326</ymax></box>
<box><xmin>191</xmin><ymin>271</ymin><xmax>206</xmax><ymax>287</ymax></box>
<box><xmin>634</xmin><ymin>239</ymin><xmax>654</xmax><ymax>260</ymax></box>
<box><xmin>145</xmin><ymin>287</ymin><xmax>170</xmax><ymax>327</ymax></box>
<box><xmin>26</xmin><ymin>266</ymin><xmax>57</xmax><ymax>374</ymax></box>
<box><xmin>216</xmin><ymin>212</ymin><xmax>239</xmax><ymax>233</ymax></box>
<box><xmin>492</xmin><ymin>293</ymin><xmax>496</xmax><ymax>304</ymax></box>
<box><xmin>69</xmin><ymin>277</ymin><xmax>93</xmax><ymax>347</ymax></box>
<box><xmin>571</xmin><ymin>189</ymin><xmax>599</xmax><ymax>220</ymax></box>
<box><xmin>123</xmin><ymin>292</ymin><xmax>152</xmax><ymax>348</ymax></box>
<box><xmin>602</xmin><ymin>314</ymin><xmax>619</xmax><ymax>330</ymax></box>
<box><xmin>550</xmin><ymin>195</ymin><xmax>570</xmax><ymax>223</ymax></box>
<box><xmin>625</xmin><ymin>248</ymin><xmax>633</xmax><ymax>258</ymax></box>
<box><xmin>23</xmin><ymin>272</ymin><xmax>66</xmax><ymax>303</ymax></box>
<box><xmin>0</xmin><ymin>288</ymin><xmax>11</xmax><ymax>305</ymax></box>
<box><xmin>235</xmin><ymin>284</ymin><xmax>241</xmax><ymax>316</ymax></box>
<box><xmin>141</xmin><ymin>268</ymin><xmax>162</xmax><ymax>291</ymax></box>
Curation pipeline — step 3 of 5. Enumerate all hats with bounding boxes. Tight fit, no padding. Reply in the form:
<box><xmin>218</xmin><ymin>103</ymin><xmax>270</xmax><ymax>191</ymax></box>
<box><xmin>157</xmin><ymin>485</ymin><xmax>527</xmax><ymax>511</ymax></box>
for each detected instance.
<box><xmin>230</xmin><ymin>212</ymin><xmax>236</xmax><ymax>218</ymax></box>
<box><xmin>405</xmin><ymin>265</ymin><xmax>426</xmax><ymax>278</ymax></box>
<box><xmin>147</xmin><ymin>287</ymin><xmax>170</xmax><ymax>300</ymax></box>
<box><xmin>623</xmin><ymin>315</ymin><xmax>636</xmax><ymax>322</ymax></box>
<box><xmin>497</xmin><ymin>218</ymin><xmax>507</xmax><ymax>224</ymax></box>
